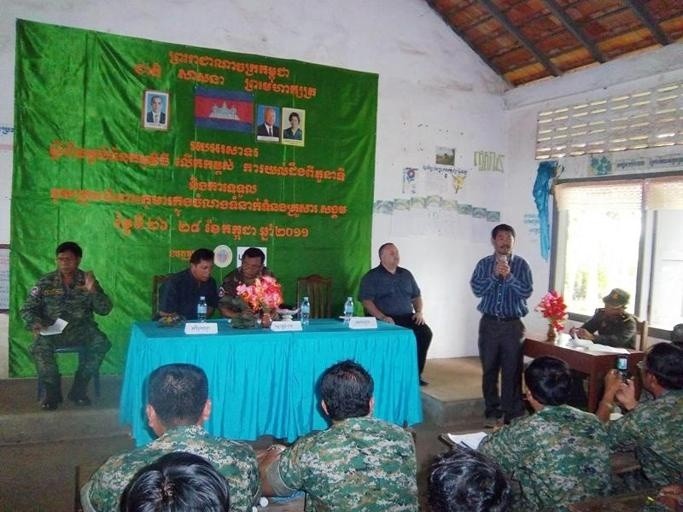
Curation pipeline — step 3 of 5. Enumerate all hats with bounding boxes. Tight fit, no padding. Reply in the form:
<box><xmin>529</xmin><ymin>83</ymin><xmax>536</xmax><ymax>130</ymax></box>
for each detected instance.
<box><xmin>602</xmin><ymin>288</ymin><xmax>630</xmax><ymax>306</ymax></box>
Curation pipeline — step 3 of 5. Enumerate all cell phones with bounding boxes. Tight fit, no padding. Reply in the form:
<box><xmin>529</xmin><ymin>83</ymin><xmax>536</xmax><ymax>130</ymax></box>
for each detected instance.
<box><xmin>615</xmin><ymin>355</ymin><xmax>629</xmax><ymax>381</ymax></box>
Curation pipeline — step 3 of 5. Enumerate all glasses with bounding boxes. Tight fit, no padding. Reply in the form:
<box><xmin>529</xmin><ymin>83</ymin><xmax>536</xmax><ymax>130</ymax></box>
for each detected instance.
<box><xmin>54</xmin><ymin>257</ymin><xmax>75</xmax><ymax>264</ymax></box>
<box><xmin>636</xmin><ymin>360</ymin><xmax>650</xmax><ymax>372</ymax></box>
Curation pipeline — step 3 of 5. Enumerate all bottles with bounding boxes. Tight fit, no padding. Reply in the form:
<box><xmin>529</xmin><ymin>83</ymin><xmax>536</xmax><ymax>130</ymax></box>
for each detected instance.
<box><xmin>196</xmin><ymin>295</ymin><xmax>206</xmax><ymax>323</ymax></box>
<box><xmin>299</xmin><ymin>297</ymin><xmax>309</xmax><ymax>325</ymax></box>
<box><xmin>343</xmin><ymin>297</ymin><xmax>353</xmax><ymax>328</ymax></box>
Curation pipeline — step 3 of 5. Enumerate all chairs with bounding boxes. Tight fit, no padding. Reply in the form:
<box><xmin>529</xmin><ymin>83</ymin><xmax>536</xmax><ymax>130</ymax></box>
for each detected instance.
<box><xmin>294</xmin><ymin>272</ymin><xmax>334</xmax><ymax>318</ymax></box>
<box><xmin>634</xmin><ymin>317</ymin><xmax>650</xmax><ymax>350</ymax></box>
<box><xmin>151</xmin><ymin>271</ymin><xmax>174</xmax><ymax>321</ymax></box>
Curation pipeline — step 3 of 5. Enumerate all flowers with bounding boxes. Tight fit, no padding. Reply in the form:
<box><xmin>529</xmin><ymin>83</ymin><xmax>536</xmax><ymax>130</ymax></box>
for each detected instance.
<box><xmin>233</xmin><ymin>274</ymin><xmax>284</xmax><ymax>313</ymax></box>
<box><xmin>532</xmin><ymin>290</ymin><xmax>569</xmax><ymax>332</ymax></box>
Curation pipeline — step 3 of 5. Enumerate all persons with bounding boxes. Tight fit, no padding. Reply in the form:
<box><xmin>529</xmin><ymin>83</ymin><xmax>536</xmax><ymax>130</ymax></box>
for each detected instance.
<box><xmin>283</xmin><ymin>111</ymin><xmax>302</xmax><ymax>140</ymax></box>
<box><xmin>594</xmin><ymin>340</ymin><xmax>683</xmax><ymax>488</ymax></box>
<box><xmin>18</xmin><ymin>240</ymin><xmax>114</xmax><ymax>410</ymax></box>
<box><xmin>669</xmin><ymin>323</ymin><xmax>683</xmax><ymax>350</ymax></box>
<box><xmin>80</xmin><ymin>362</ymin><xmax>263</xmax><ymax>511</ymax></box>
<box><xmin>256</xmin><ymin>106</ymin><xmax>280</xmax><ymax>137</ymax></box>
<box><xmin>357</xmin><ymin>242</ymin><xmax>433</xmax><ymax>386</ymax></box>
<box><xmin>569</xmin><ymin>287</ymin><xmax>638</xmax><ymax>414</ymax></box>
<box><xmin>469</xmin><ymin>223</ymin><xmax>534</xmax><ymax>427</ymax></box>
<box><xmin>159</xmin><ymin>248</ymin><xmax>219</xmax><ymax>320</ymax></box>
<box><xmin>146</xmin><ymin>95</ymin><xmax>166</xmax><ymax>124</ymax></box>
<box><xmin>117</xmin><ymin>451</ymin><xmax>230</xmax><ymax>511</ymax></box>
<box><xmin>217</xmin><ymin>246</ymin><xmax>280</xmax><ymax>318</ymax></box>
<box><xmin>656</xmin><ymin>483</ymin><xmax>683</xmax><ymax>511</ymax></box>
<box><xmin>476</xmin><ymin>355</ymin><xmax>618</xmax><ymax>510</ymax></box>
<box><xmin>255</xmin><ymin>358</ymin><xmax>425</xmax><ymax>512</ymax></box>
<box><xmin>424</xmin><ymin>446</ymin><xmax>513</xmax><ymax>512</ymax></box>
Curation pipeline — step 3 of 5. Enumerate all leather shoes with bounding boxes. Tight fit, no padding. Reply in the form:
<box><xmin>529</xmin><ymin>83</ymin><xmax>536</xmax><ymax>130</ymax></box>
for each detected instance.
<box><xmin>67</xmin><ymin>392</ymin><xmax>91</xmax><ymax>407</ymax></box>
<box><xmin>41</xmin><ymin>399</ymin><xmax>58</xmax><ymax>410</ymax></box>
<box><xmin>419</xmin><ymin>374</ymin><xmax>430</xmax><ymax>385</ymax></box>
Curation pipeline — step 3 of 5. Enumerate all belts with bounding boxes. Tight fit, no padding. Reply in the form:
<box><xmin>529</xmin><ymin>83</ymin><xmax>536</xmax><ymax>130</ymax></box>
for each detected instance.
<box><xmin>484</xmin><ymin>313</ymin><xmax>519</xmax><ymax>321</ymax></box>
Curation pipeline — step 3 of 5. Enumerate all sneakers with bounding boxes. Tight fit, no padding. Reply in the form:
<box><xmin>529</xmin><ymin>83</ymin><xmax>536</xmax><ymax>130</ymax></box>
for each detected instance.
<box><xmin>483</xmin><ymin>416</ymin><xmax>497</xmax><ymax>427</ymax></box>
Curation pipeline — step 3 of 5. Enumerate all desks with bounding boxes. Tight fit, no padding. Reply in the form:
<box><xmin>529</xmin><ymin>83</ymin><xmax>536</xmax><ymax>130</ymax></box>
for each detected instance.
<box><xmin>565</xmin><ymin>486</ymin><xmax>671</xmax><ymax>511</ymax></box>
<box><xmin>75</xmin><ymin>441</ymin><xmax>306</xmax><ymax>512</ymax></box>
<box><xmin>438</xmin><ymin>424</ymin><xmax>647</xmax><ymax>479</ymax></box>
<box><xmin>518</xmin><ymin>334</ymin><xmax>648</xmax><ymax>416</ymax></box>
<box><xmin>121</xmin><ymin>317</ymin><xmax>426</xmax><ymax>450</ymax></box>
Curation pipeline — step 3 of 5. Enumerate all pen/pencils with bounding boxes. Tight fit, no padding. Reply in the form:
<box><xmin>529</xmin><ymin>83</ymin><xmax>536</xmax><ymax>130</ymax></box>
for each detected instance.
<box><xmin>461</xmin><ymin>441</ymin><xmax>473</xmax><ymax>449</ymax></box>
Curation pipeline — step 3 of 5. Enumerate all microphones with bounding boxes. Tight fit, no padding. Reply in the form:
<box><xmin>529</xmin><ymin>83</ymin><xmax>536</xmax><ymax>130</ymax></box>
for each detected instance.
<box><xmin>498</xmin><ymin>254</ymin><xmax>507</xmax><ymax>286</ymax></box>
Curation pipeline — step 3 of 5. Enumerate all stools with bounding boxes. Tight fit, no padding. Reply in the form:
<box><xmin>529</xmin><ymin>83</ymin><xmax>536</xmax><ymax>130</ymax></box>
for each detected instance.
<box><xmin>32</xmin><ymin>343</ymin><xmax>101</xmax><ymax>402</ymax></box>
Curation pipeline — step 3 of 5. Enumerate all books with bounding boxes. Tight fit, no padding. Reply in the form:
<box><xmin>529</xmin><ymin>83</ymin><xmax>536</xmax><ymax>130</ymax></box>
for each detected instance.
<box><xmin>38</xmin><ymin>316</ymin><xmax>69</xmax><ymax>335</ymax></box>
<box><xmin>566</xmin><ymin>330</ymin><xmax>632</xmax><ymax>355</ymax></box>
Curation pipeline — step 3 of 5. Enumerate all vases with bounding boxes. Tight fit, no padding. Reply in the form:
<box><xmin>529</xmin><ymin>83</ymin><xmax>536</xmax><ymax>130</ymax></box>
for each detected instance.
<box><xmin>546</xmin><ymin>323</ymin><xmax>558</xmax><ymax>341</ymax></box>
<box><xmin>253</xmin><ymin>304</ymin><xmax>273</xmax><ymax>330</ymax></box>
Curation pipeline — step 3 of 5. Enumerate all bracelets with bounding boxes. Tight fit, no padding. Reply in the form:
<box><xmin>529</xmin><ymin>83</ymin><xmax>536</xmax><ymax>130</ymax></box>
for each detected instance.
<box><xmin>598</xmin><ymin>399</ymin><xmax>616</xmax><ymax>414</ymax></box>
<box><xmin>265</xmin><ymin>444</ymin><xmax>286</xmax><ymax>451</ymax></box>
